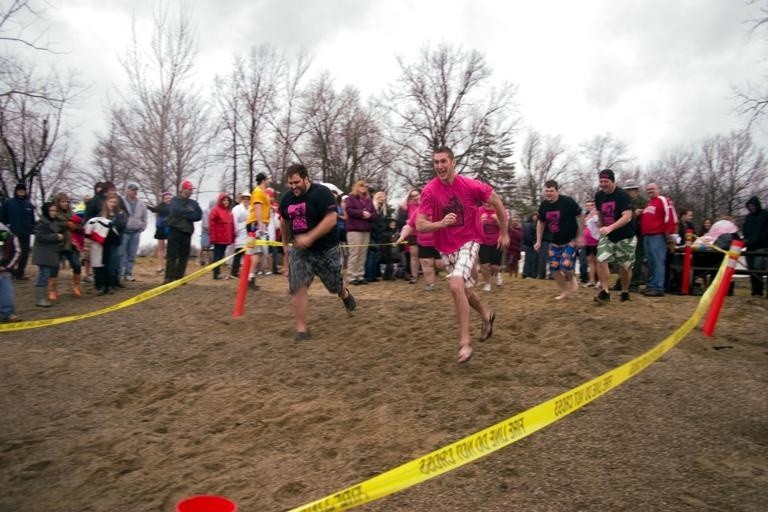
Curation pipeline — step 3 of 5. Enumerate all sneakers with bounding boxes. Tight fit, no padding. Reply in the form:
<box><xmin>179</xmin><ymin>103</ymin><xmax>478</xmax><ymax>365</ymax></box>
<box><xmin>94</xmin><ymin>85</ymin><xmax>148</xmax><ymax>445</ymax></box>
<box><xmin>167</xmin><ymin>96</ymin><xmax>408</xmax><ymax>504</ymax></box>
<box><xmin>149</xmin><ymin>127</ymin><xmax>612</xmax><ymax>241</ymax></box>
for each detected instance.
<box><xmin>479</xmin><ymin>306</ymin><xmax>496</xmax><ymax>340</ymax></box>
<box><xmin>341</xmin><ymin>286</ymin><xmax>356</xmax><ymax>312</ymax></box>
<box><xmin>456</xmin><ymin>336</ymin><xmax>474</xmax><ymax>363</ymax></box>
<box><xmin>582</xmin><ymin>279</ymin><xmax>666</xmax><ymax>304</ymax></box>
<box><xmin>83</xmin><ymin>273</ymin><xmax>135</xmax><ymax>295</ymax></box>
<box><xmin>289</xmin><ymin>327</ymin><xmax>313</xmax><ymax>347</ymax></box>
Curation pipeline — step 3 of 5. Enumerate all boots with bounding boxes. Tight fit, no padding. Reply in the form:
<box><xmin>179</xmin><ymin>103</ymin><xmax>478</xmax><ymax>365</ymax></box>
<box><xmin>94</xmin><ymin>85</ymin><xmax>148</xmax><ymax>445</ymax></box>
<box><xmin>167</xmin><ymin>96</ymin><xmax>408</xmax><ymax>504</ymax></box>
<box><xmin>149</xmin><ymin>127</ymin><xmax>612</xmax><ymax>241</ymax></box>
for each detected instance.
<box><xmin>35</xmin><ymin>274</ymin><xmax>62</xmax><ymax>307</ymax></box>
<box><xmin>71</xmin><ymin>272</ymin><xmax>82</xmax><ymax>297</ymax></box>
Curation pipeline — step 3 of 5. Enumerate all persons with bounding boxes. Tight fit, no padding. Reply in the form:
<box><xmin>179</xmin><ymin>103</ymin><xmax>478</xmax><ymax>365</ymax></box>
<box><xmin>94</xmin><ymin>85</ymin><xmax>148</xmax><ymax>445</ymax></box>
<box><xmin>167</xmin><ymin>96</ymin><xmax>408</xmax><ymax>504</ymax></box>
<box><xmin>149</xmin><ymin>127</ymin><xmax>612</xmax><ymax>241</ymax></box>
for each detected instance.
<box><xmin>2</xmin><ymin>182</ymin><xmax>147</xmax><ymax>322</ymax></box>
<box><xmin>280</xmin><ymin>164</ymin><xmax>358</xmax><ymax>346</ymax></box>
<box><xmin>196</xmin><ymin>171</ymin><xmax>289</xmax><ymax>291</ymax></box>
<box><xmin>471</xmin><ymin>168</ymin><xmax>678</xmax><ymax>304</ymax></box>
<box><xmin>415</xmin><ymin>147</ymin><xmax>513</xmax><ymax>365</ymax></box>
<box><xmin>146</xmin><ymin>181</ymin><xmax>202</xmax><ymax>286</ymax></box>
<box><xmin>676</xmin><ymin>210</ymin><xmax>734</xmax><ymax>244</ymax></box>
<box><xmin>319</xmin><ymin>181</ymin><xmax>447</xmax><ymax>291</ymax></box>
<box><xmin>742</xmin><ymin>195</ymin><xmax>766</xmax><ymax>296</ymax></box>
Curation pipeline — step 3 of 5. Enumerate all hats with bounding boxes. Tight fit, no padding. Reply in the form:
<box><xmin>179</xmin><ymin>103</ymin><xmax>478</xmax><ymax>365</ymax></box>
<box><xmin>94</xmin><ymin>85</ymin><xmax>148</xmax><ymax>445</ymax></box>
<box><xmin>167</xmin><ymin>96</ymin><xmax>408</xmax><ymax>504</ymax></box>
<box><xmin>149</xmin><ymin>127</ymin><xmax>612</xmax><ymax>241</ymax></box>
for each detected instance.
<box><xmin>621</xmin><ymin>180</ymin><xmax>639</xmax><ymax>189</ymax></box>
<box><xmin>255</xmin><ymin>172</ymin><xmax>273</xmax><ymax>182</ymax></box>
<box><xmin>127</xmin><ymin>184</ymin><xmax>138</xmax><ymax>190</ymax></box>
<box><xmin>181</xmin><ymin>180</ymin><xmax>197</xmax><ymax>190</ymax></box>
